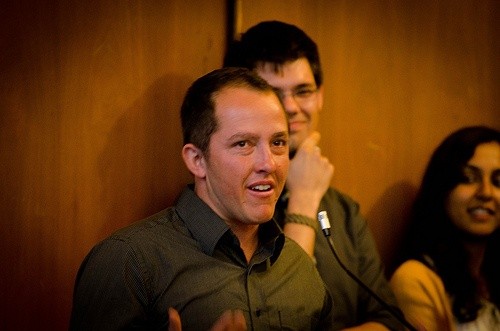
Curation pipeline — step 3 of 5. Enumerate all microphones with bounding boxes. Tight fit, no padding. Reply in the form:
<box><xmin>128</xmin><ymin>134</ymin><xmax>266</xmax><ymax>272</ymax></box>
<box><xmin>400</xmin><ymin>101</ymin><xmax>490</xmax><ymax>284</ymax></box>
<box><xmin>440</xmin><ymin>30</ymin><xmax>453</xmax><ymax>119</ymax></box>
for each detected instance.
<box><xmin>318</xmin><ymin>210</ymin><xmax>418</xmax><ymax>331</ymax></box>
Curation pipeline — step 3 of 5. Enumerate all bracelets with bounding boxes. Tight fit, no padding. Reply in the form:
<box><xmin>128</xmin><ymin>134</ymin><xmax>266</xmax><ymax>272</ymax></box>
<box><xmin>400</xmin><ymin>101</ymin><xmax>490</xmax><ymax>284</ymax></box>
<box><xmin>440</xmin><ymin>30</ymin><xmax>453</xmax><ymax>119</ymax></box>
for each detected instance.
<box><xmin>285</xmin><ymin>214</ymin><xmax>319</xmax><ymax>231</ymax></box>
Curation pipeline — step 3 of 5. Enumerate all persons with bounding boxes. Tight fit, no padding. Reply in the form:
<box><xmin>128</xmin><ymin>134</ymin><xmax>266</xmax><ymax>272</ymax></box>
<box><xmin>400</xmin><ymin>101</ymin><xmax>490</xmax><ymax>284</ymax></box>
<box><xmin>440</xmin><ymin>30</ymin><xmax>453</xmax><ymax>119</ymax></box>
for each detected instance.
<box><xmin>70</xmin><ymin>67</ymin><xmax>333</xmax><ymax>331</ymax></box>
<box><xmin>223</xmin><ymin>19</ymin><xmax>403</xmax><ymax>331</ymax></box>
<box><xmin>390</xmin><ymin>125</ymin><xmax>500</xmax><ymax>331</ymax></box>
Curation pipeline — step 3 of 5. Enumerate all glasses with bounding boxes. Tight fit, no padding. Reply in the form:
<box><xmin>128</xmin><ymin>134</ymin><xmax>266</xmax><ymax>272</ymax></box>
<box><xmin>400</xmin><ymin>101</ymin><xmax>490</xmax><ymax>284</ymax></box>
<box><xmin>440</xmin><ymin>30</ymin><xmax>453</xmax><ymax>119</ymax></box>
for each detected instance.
<box><xmin>277</xmin><ymin>86</ymin><xmax>319</xmax><ymax>103</ymax></box>
